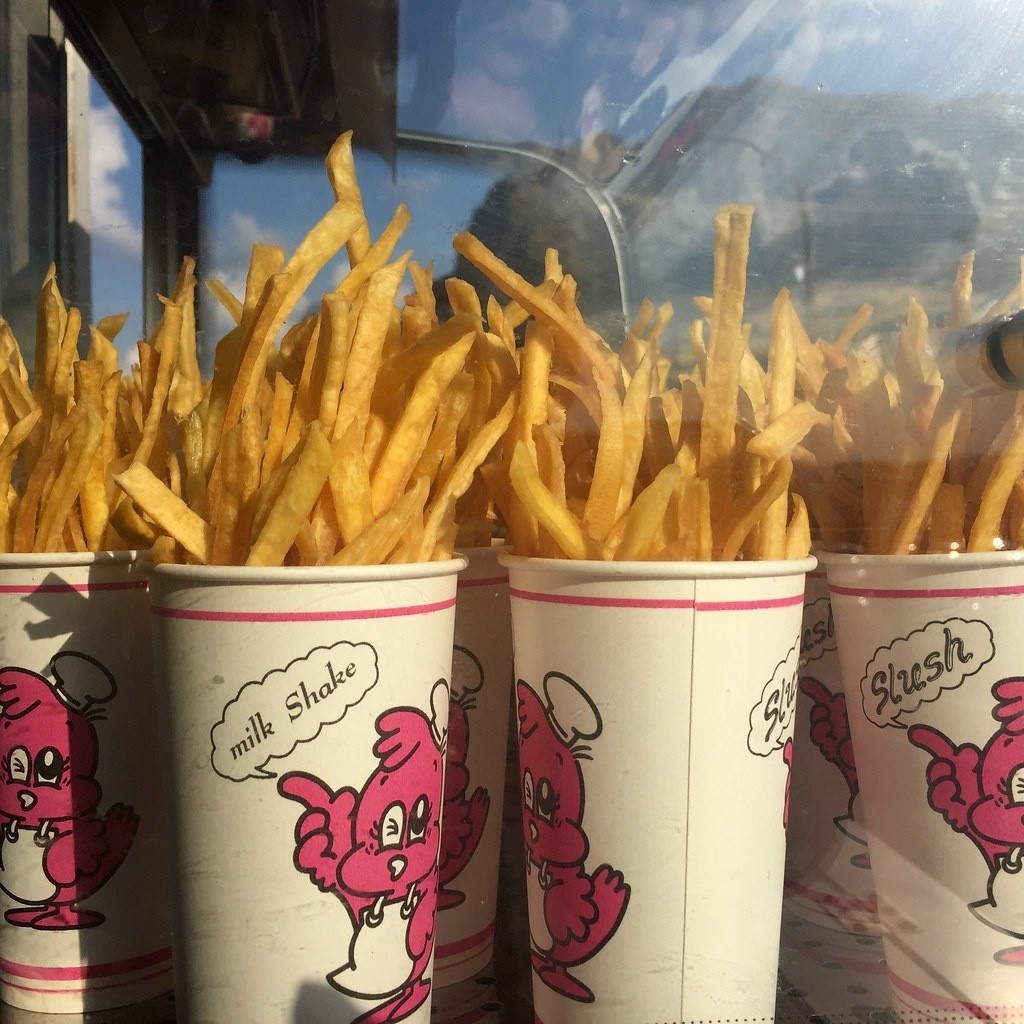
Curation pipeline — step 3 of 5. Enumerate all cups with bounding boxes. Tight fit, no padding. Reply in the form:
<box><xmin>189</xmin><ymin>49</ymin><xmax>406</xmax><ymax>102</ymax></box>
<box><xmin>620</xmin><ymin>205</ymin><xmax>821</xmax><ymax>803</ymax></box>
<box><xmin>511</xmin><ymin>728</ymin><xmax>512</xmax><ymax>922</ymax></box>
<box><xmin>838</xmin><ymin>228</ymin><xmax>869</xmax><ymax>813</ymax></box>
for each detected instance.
<box><xmin>788</xmin><ymin>546</ymin><xmax>1024</xmax><ymax>1021</ymax></box>
<box><xmin>0</xmin><ymin>551</ymin><xmax>181</xmax><ymax>1013</ymax></box>
<box><xmin>140</xmin><ymin>548</ymin><xmax>469</xmax><ymax>1024</ymax></box>
<box><xmin>433</xmin><ymin>537</ymin><xmax>517</xmax><ymax>985</ymax></box>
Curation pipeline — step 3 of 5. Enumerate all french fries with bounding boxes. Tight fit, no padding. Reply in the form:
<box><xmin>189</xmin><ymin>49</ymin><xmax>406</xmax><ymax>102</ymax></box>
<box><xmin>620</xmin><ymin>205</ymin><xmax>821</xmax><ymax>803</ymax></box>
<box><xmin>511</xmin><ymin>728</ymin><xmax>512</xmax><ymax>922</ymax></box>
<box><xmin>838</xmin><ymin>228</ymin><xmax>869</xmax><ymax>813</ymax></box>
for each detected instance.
<box><xmin>0</xmin><ymin>130</ymin><xmax>1023</xmax><ymax>570</ymax></box>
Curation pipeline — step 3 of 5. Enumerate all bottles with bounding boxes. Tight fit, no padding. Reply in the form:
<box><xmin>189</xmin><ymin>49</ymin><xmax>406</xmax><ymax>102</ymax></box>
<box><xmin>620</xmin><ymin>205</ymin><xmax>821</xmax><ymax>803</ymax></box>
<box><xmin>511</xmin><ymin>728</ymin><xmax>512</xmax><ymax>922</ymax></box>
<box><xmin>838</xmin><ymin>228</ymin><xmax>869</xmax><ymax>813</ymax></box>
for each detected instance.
<box><xmin>495</xmin><ymin>543</ymin><xmax>818</xmax><ymax>1023</ymax></box>
